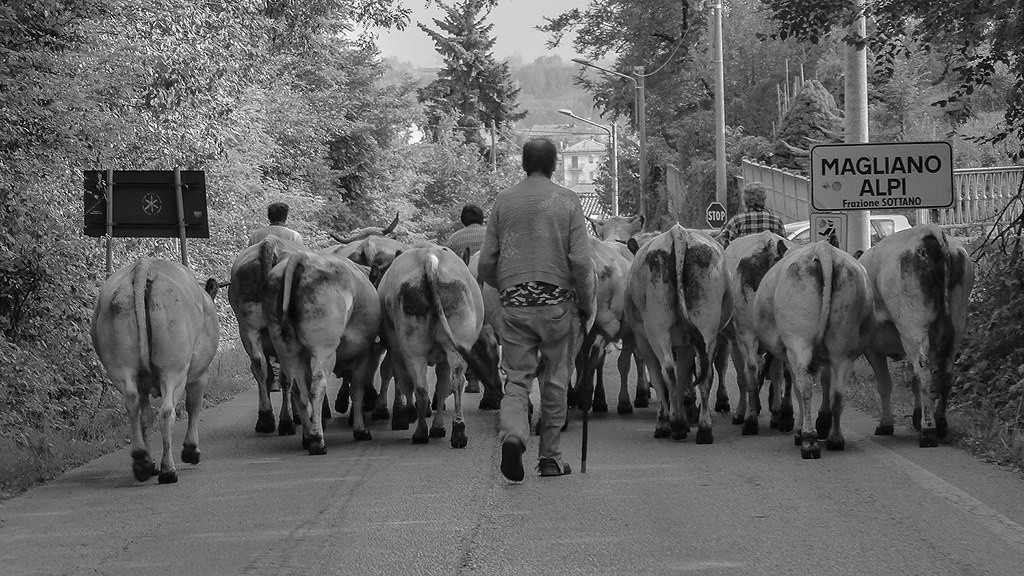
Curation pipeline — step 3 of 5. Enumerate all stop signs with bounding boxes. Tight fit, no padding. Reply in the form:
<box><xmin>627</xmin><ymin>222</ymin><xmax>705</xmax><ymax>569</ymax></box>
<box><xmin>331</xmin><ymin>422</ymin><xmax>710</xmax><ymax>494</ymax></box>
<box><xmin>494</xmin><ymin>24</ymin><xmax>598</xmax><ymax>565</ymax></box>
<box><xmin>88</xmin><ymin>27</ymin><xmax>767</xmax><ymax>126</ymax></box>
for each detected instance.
<box><xmin>706</xmin><ymin>202</ymin><xmax>728</xmax><ymax>230</ymax></box>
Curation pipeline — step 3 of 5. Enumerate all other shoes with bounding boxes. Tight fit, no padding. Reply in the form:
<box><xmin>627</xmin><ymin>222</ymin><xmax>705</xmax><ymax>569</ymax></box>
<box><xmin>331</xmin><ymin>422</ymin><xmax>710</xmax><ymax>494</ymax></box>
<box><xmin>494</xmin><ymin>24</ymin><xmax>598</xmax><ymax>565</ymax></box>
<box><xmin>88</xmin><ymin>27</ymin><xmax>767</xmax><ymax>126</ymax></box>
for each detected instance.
<box><xmin>500</xmin><ymin>438</ymin><xmax>524</xmax><ymax>481</ymax></box>
<box><xmin>539</xmin><ymin>457</ymin><xmax>571</xmax><ymax>477</ymax></box>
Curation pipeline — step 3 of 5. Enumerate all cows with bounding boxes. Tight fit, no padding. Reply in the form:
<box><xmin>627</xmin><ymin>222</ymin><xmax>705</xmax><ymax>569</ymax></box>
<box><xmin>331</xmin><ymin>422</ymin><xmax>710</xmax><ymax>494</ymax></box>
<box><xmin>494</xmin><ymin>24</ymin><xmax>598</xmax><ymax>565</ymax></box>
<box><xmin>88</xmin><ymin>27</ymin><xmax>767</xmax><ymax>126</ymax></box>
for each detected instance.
<box><xmin>228</xmin><ymin>210</ymin><xmax>975</xmax><ymax>461</ymax></box>
<box><xmin>88</xmin><ymin>255</ymin><xmax>232</xmax><ymax>485</ymax></box>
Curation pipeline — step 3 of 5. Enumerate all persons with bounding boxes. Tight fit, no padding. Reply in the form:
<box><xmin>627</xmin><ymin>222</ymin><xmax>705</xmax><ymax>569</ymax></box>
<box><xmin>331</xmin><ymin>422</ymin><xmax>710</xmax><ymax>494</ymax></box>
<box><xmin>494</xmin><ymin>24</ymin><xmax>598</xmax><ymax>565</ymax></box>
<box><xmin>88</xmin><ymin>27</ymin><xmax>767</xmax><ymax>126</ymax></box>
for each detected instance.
<box><xmin>714</xmin><ymin>182</ymin><xmax>788</xmax><ymax>250</ymax></box>
<box><xmin>445</xmin><ymin>205</ymin><xmax>487</xmax><ymax>393</ymax></box>
<box><xmin>478</xmin><ymin>139</ymin><xmax>595</xmax><ymax>482</ymax></box>
<box><xmin>248</xmin><ymin>202</ymin><xmax>304</xmax><ymax>391</ymax></box>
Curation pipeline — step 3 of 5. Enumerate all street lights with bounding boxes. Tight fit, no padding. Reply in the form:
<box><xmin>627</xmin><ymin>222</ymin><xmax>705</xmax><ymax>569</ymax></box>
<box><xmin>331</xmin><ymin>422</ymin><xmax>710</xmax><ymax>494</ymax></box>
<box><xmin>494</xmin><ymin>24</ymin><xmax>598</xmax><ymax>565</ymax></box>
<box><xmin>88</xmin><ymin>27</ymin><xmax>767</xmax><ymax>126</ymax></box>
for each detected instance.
<box><xmin>571</xmin><ymin>57</ymin><xmax>648</xmax><ymax>217</ymax></box>
<box><xmin>558</xmin><ymin>109</ymin><xmax>619</xmax><ymax>218</ymax></box>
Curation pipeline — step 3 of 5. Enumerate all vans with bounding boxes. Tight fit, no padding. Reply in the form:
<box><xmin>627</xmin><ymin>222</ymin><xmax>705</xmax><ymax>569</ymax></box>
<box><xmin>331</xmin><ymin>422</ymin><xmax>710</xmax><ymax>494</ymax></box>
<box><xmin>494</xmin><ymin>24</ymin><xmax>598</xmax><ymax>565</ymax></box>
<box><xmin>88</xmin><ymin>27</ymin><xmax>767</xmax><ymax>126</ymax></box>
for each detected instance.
<box><xmin>783</xmin><ymin>215</ymin><xmax>912</xmax><ymax>248</ymax></box>
